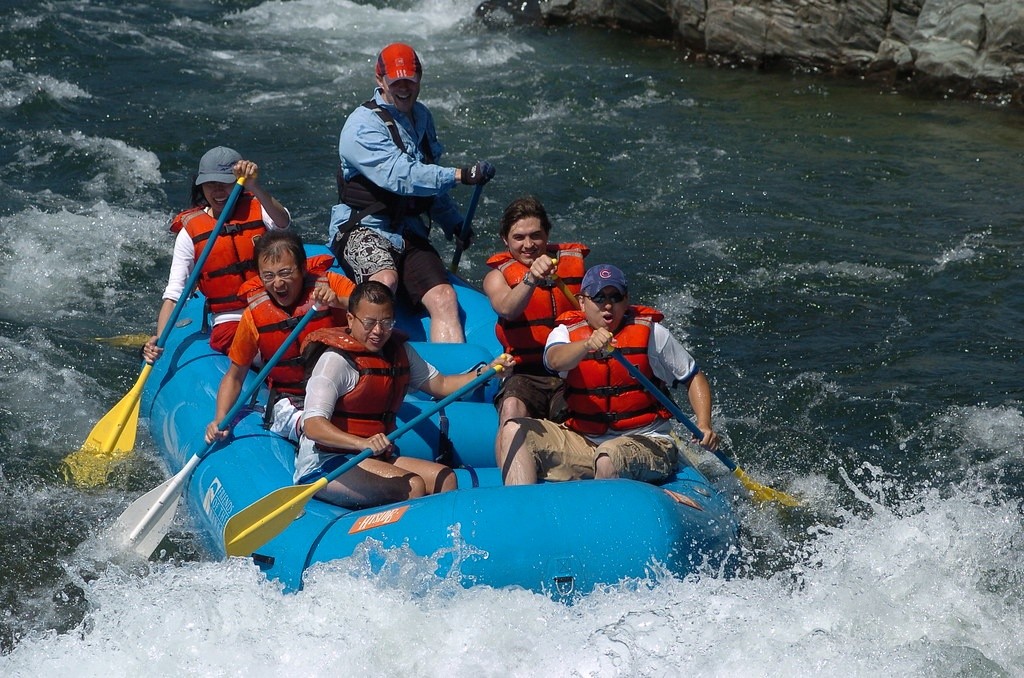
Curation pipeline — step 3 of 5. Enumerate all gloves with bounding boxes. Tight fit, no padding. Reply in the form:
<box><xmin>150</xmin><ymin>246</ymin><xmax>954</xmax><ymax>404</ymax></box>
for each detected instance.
<box><xmin>460</xmin><ymin>163</ymin><xmax>498</xmax><ymax>185</ymax></box>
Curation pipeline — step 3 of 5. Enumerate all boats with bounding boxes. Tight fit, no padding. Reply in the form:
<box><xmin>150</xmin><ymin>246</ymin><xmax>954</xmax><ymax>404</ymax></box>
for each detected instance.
<box><xmin>137</xmin><ymin>238</ymin><xmax>740</xmax><ymax>614</ymax></box>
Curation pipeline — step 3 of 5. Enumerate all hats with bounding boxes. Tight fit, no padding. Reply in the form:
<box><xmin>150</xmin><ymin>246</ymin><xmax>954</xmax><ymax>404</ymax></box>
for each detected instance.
<box><xmin>580</xmin><ymin>264</ymin><xmax>628</xmax><ymax>298</ymax></box>
<box><xmin>376</xmin><ymin>42</ymin><xmax>424</xmax><ymax>86</ymax></box>
<box><xmin>194</xmin><ymin>144</ymin><xmax>244</xmax><ymax>187</ymax></box>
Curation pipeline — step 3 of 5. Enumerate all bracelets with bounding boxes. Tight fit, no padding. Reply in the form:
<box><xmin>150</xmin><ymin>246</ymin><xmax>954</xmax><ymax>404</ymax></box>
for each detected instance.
<box><xmin>477</xmin><ymin>368</ymin><xmax>489</xmax><ymax>386</ymax></box>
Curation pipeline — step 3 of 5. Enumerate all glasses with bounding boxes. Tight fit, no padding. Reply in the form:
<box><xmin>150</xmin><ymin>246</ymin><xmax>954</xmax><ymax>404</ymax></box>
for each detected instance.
<box><xmin>583</xmin><ymin>290</ymin><xmax>629</xmax><ymax>305</ymax></box>
<box><xmin>260</xmin><ymin>266</ymin><xmax>301</xmax><ymax>283</ymax></box>
<box><xmin>352</xmin><ymin>313</ymin><xmax>397</xmax><ymax>330</ymax></box>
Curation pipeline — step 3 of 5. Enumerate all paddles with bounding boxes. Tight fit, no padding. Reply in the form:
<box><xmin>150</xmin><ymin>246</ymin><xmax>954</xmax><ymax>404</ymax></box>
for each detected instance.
<box><xmin>222</xmin><ymin>354</ymin><xmax>508</xmax><ymax>561</ymax></box>
<box><xmin>101</xmin><ymin>302</ymin><xmax>322</xmax><ymax>561</ymax></box>
<box><xmin>450</xmin><ymin>181</ymin><xmax>482</xmax><ymax>275</ymax></box>
<box><xmin>62</xmin><ymin>166</ymin><xmax>257</xmax><ymax>490</ymax></box>
<box><xmin>546</xmin><ymin>258</ymin><xmax>583</xmax><ymax>313</ymax></box>
<box><xmin>604</xmin><ymin>332</ymin><xmax>799</xmax><ymax>510</ymax></box>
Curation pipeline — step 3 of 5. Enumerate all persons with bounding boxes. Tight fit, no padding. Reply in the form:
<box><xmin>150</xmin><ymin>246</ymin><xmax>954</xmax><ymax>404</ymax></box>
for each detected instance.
<box><xmin>144</xmin><ymin>147</ymin><xmax>718</xmax><ymax>507</ymax></box>
<box><xmin>327</xmin><ymin>44</ymin><xmax>494</xmax><ymax>342</ymax></box>
